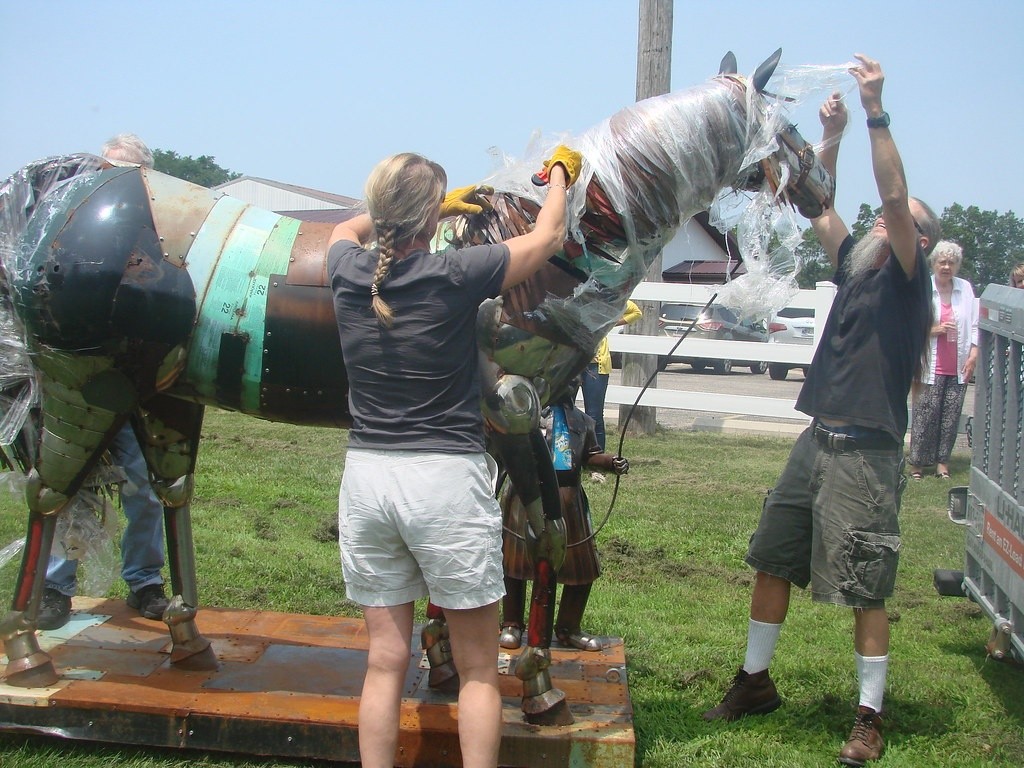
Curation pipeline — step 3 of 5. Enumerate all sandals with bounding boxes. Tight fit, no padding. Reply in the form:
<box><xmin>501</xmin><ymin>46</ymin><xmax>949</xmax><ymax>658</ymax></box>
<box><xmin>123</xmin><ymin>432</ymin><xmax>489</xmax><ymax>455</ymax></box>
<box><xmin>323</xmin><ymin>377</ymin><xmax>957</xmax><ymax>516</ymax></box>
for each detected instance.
<box><xmin>911</xmin><ymin>472</ymin><xmax>922</xmax><ymax>481</ymax></box>
<box><xmin>936</xmin><ymin>473</ymin><xmax>948</xmax><ymax>479</ymax></box>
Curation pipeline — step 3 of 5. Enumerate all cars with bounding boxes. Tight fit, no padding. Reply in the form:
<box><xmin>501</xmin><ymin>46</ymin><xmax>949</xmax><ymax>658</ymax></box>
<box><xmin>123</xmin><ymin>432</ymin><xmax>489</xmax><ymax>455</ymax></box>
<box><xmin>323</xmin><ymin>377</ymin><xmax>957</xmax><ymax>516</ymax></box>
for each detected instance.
<box><xmin>768</xmin><ymin>308</ymin><xmax>814</xmax><ymax>381</ymax></box>
<box><xmin>657</xmin><ymin>302</ymin><xmax>770</xmax><ymax>376</ymax></box>
<box><xmin>604</xmin><ymin>325</ymin><xmax>625</xmax><ymax>369</ymax></box>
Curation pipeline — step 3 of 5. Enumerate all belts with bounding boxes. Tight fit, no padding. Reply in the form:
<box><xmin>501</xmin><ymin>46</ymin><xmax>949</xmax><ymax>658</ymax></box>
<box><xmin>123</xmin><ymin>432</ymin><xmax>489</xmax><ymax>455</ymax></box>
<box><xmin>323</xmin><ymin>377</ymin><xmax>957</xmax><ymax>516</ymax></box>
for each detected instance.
<box><xmin>811</xmin><ymin>418</ymin><xmax>893</xmax><ymax>452</ymax></box>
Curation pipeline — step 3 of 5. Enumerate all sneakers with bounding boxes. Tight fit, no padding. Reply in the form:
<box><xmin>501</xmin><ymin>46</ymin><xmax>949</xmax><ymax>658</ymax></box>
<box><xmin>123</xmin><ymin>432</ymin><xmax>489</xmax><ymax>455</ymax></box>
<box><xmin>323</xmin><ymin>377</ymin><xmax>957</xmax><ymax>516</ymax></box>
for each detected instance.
<box><xmin>838</xmin><ymin>705</ymin><xmax>884</xmax><ymax>766</ymax></box>
<box><xmin>127</xmin><ymin>582</ymin><xmax>171</xmax><ymax>619</ymax></box>
<box><xmin>35</xmin><ymin>588</ymin><xmax>71</xmax><ymax>630</ymax></box>
<box><xmin>705</xmin><ymin>668</ymin><xmax>782</xmax><ymax>725</ymax></box>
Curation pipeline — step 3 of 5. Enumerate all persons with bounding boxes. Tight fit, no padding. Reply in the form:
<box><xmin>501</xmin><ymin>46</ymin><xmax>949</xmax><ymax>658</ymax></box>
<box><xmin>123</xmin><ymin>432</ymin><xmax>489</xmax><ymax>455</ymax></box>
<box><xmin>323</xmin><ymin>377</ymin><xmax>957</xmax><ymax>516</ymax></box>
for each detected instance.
<box><xmin>33</xmin><ymin>134</ymin><xmax>171</xmax><ymax>631</ymax></box>
<box><xmin>989</xmin><ymin>262</ymin><xmax>1024</xmax><ymax>383</ymax></box>
<box><xmin>580</xmin><ymin>299</ymin><xmax>642</xmax><ymax>482</ymax></box>
<box><xmin>326</xmin><ymin>145</ymin><xmax>583</xmax><ymax>768</ymax></box>
<box><xmin>701</xmin><ymin>52</ymin><xmax>941</xmax><ymax>768</ymax></box>
<box><xmin>498</xmin><ymin>372</ymin><xmax>629</xmax><ymax>652</ymax></box>
<box><xmin>907</xmin><ymin>239</ymin><xmax>978</xmax><ymax>481</ymax></box>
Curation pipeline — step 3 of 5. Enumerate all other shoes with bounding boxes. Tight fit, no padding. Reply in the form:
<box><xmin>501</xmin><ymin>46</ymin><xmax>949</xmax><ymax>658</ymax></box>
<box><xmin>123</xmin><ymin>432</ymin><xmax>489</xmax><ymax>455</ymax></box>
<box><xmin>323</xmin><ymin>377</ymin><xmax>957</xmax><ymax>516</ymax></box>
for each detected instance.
<box><xmin>591</xmin><ymin>472</ymin><xmax>605</xmax><ymax>484</ymax></box>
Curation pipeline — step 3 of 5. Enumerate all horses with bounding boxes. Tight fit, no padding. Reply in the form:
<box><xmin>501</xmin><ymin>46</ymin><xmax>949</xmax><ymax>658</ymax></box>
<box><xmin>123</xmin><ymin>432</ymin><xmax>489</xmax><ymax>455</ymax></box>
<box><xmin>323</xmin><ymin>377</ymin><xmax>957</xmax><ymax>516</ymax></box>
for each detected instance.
<box><xmin>0</xmin><ymin>48</ymin><xmax>835</xmax><ymax>727</ymax></box>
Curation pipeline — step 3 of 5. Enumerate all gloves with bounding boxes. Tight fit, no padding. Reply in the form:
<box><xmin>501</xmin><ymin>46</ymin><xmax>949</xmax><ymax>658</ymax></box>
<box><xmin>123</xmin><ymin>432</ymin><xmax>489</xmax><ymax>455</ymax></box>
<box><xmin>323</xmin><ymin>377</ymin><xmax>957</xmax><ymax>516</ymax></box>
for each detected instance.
<box><xmin>435</xmin><ymin>185</ymin><xmax>495</xmax><ymax>222</ymax></box>
<box><xmin>544</xmin><ymin>144</ymin><xmax>582</xmax><ymax>190</ymax></box>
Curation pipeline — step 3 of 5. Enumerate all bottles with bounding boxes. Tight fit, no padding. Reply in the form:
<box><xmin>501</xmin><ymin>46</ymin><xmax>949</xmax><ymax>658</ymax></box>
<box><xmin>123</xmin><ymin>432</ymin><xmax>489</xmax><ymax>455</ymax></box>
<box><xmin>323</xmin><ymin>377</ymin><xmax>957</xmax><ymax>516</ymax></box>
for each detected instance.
<box><xmin>947</xmin><ymin>313</ymin><xmax>957</xmax><ymax>342</ymax></box>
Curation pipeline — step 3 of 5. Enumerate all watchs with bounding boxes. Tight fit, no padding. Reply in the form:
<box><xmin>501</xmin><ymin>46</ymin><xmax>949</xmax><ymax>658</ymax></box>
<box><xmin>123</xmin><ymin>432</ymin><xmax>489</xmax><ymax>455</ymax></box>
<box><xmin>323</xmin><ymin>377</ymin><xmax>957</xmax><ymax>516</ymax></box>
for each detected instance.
<box><xmin>867</xmin><ymin>111</ymin><xmax>890</xmax><ymax>128</ymax></box>
